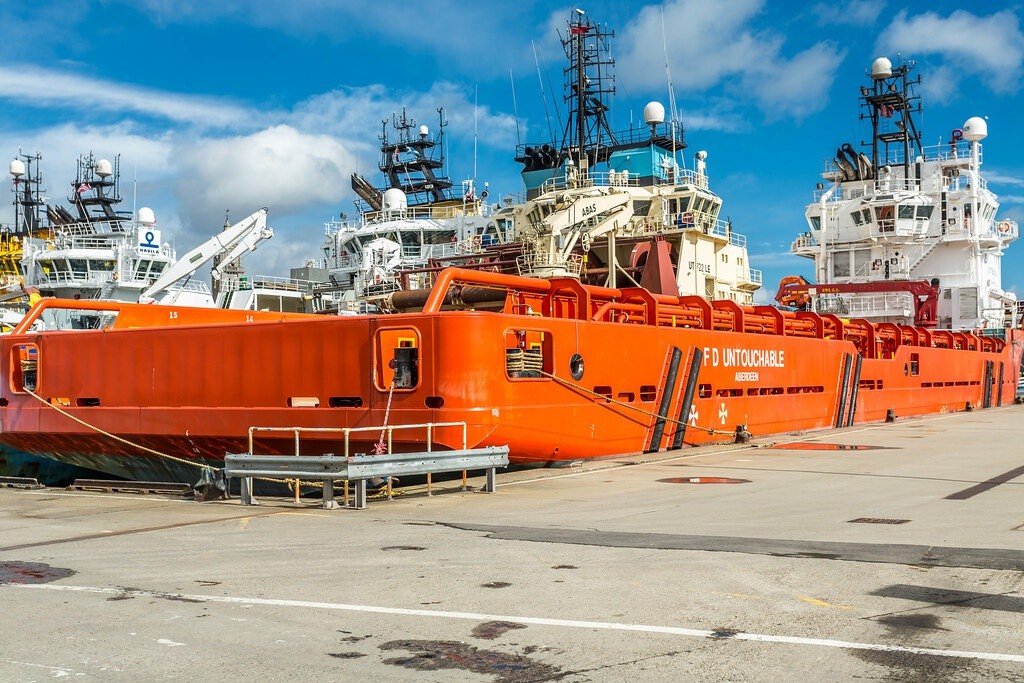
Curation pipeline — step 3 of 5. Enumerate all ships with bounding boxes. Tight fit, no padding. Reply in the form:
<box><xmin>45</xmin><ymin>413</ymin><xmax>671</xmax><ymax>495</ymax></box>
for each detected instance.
<box><xmin>0</xmin><ymin>1</ymin><xmax>1024</xmax><ymax>511</ymax></box>
